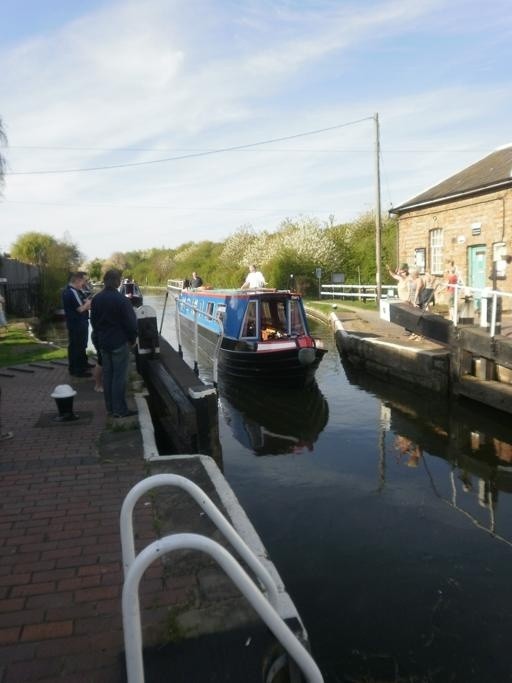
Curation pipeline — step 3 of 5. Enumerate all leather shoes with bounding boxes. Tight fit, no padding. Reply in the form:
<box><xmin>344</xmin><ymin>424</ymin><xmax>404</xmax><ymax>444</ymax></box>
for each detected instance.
<box><xmin>69</xmin><ymin>363</ymin><xmax>97</xmax><ymax>378</ymax></box>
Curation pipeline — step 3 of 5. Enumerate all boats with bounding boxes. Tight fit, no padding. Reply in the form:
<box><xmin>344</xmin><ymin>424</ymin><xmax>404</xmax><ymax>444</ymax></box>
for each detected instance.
<box><xmin>219</xmin><ymin>378</ymin><xmax>330</xmax><ymax>455</ymax></box>
<box><xmin>167</xmin><ymin>266</ymin><xmax>328</xmax><ymax>387</ymax></box>
<box><xmin>118</xmin><ymin>277</ymin><xmax>143</xmax><ymax>304</ymax></box>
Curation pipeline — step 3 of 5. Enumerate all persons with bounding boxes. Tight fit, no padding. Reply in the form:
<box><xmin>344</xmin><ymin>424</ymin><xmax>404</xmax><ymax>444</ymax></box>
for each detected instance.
<box><xmin>189</xmin><ymin>270</ymin><xmax>203</xmax><ymax>290</ymax></box>
<box><xmin>405</xmin><ymin>267</ymin><xmax>426</xmax><ymax>341</ymax></box>
<box><xmin>90</xmin><ymin>267</ymin><xmax>138</xmax><ymax>417</ymax></box>
<box><xmin>240</xmin><ymin>263</ymin><xmax>268</xmax><ymax>323</ymax></box>
<box><xmin>437</xmin><ymin>267</ymin><xmax>460</xmax><ymax>320</ymax></box>
<box><xmin>78</xmin><ymin>271</ymin><xmax>97</xmax><ymax>366</ymax></box>
<box><xmin>64</xmin><ymin>270</ymin><xmax>94</xmax><ymax>377</ymax></box>
<box><xmin>386</xmin><ymin>261</ymin><xmax>412</xmax><ymax>335</ymax></box>
<box><xmin>91</xmin><ymin>329</ymin><xmax>104</xmax><ymax>393</ymax></box>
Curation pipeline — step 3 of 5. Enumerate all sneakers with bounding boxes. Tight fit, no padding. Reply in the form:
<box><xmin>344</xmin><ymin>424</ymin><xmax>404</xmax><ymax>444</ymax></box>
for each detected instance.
<box><xmin>93</xmin><ymin>384</ymin><xmax>105</xmax><ymax>393</ymax></box>
<box><xmin>408</xmin><ymin>332</ymin><xmax>425</xmax><ymax>342</ymax></box>
<box><xmin>105</xmin><ymin>409</ymin><xmax>138</xmax><ymax>418</ymax></box>
